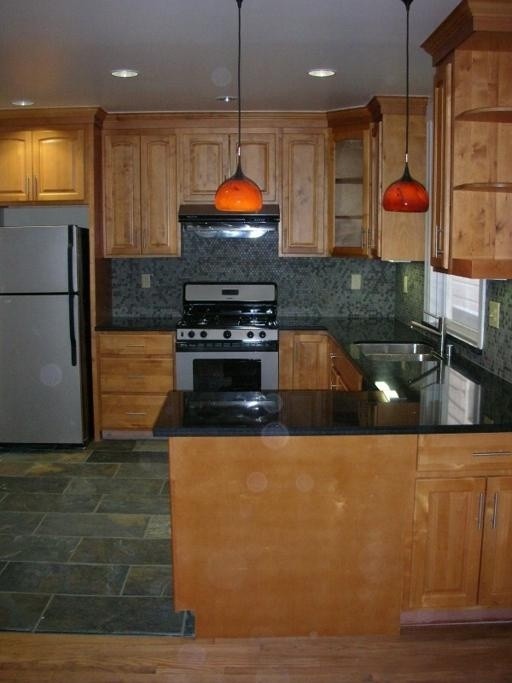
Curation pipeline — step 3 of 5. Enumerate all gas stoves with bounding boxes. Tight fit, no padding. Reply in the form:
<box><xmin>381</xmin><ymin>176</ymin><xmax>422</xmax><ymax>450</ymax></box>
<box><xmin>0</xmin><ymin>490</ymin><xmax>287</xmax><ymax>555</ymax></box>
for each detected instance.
<box><xmin>182</xmin><ymin>304</ymin><xmax>277</xmax><ymax>326</ymax></box>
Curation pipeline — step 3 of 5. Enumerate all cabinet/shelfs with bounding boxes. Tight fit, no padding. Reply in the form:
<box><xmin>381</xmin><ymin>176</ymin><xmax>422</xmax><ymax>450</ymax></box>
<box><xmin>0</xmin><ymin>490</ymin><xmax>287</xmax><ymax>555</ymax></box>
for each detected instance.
<box><xmin>419</xmin><ymin>0</ymin><xmax>512</xmax><ymax>279</ymax></box>
<box><xmin>328</xmin><ymin>95</ymin><xmax>428</xmax><ymax>262</ymax></box>
<box><xmin>328</xmin><ymin>330</ymin><xmax>363</xmax><ymax>390</ymax></box>
<box><xmin>279</xmin><ymin>329</ymin><xmax>328</xmax><ymax>390</ymax></box>
<box><xmin>400</xmin><ymin>432</ymin><xmax>512</xmax><ymax>627</ymax></box>
<box><xmin>0</xmin><ymin>108</ymin><xmax>89</xmax><ymax>207</ymax></box>
<box><xmin>278</xmin><ymin>112</ymin><xmax>328</xmax><ymax>257</ymax></box>
<box><xmin>180</xmin><ymin>112</ymin><xmax>279</xmax><ymax>205</ymax></box>
<box><xmin>97</xmin><ymin>331</ymin><xmax>176</xmax><ymax>440</ymax></box>
<box><xmin>102</xmin><ymin>112</ymin><xmax>182</xmax><ymax>258</ymax></box>
<box><xmin>278</xmin><ymin>390</ymin><xmax>420</xmax><ymax>430</ymax></box>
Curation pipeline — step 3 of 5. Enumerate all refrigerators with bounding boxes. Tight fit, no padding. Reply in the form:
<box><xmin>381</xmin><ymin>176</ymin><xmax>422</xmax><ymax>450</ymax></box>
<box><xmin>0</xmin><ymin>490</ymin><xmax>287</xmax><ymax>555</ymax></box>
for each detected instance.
<box><xmin>0</xmin><ymin>223</ymin><xmax>90</xmax><ymax>445</ymax></box>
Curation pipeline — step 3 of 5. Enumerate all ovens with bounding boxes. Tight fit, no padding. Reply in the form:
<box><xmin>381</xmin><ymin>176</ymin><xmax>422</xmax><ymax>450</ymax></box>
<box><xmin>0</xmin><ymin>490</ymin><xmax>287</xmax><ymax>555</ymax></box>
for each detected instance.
<box><xmin>176</xmin><ymin>340</ymin><xmax>279</xmax><ymax>390</ymax></box>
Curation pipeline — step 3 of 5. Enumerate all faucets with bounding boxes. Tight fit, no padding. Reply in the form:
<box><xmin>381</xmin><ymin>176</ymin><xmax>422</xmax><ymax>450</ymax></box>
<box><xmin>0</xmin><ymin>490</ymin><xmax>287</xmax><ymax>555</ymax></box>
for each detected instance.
<box><xmin>408</xmin><ymin>311</ymin><xmax>446</xmax><ymax>355</ymax></box>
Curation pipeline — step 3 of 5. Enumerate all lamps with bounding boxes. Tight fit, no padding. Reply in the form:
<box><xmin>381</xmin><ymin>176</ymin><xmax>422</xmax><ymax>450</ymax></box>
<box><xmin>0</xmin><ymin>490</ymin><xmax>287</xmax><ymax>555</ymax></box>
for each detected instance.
<box><xmin>214</xmin><ymin>0</ymin><xmax>263</xmax><ymax>213</ymax></box>
<box><xmin>382</xmin><ymin>0</ymin><xmax>429</xmax><ymax>213</ymax></box>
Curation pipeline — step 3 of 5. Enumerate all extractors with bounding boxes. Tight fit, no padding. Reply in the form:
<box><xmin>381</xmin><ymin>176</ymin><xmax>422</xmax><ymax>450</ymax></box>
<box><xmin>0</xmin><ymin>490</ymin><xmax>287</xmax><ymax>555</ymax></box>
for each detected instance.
<box><xmin>179</xmin><ymin>203</ymin><xmax>280</xmax><ymax>226</ymax></box>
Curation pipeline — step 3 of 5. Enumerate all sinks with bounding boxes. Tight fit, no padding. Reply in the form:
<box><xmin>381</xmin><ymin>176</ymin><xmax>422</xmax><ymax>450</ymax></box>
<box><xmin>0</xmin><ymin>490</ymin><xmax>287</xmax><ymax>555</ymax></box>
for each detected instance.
<box><xmin>362</xmin><ymin>354</ymin><xmax>444</xmax><ymax>361</ymax></box>
<box><xmin>353</xmin><ymin>340</ymin><xmax>444</xmax><ymax>354</ymax></box>
